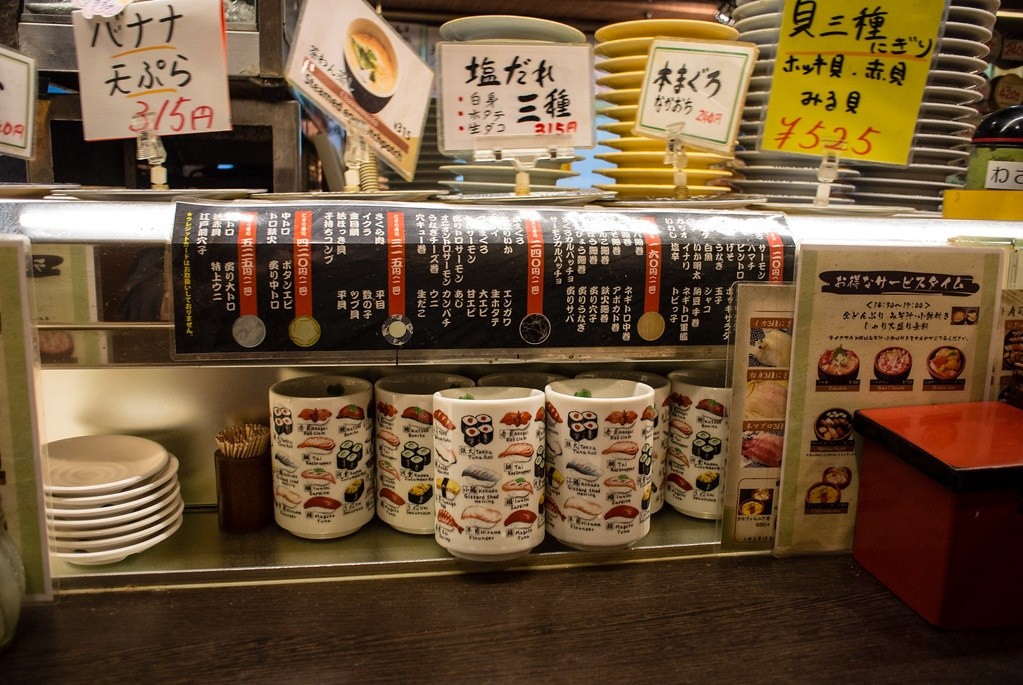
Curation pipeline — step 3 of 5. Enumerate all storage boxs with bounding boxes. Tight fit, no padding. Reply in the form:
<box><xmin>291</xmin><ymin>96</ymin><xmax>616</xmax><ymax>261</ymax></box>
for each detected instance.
<box><xmin>848</xmin><ymin>402</ymin><xmax>1023</xmax><ymax>634</ymax></box>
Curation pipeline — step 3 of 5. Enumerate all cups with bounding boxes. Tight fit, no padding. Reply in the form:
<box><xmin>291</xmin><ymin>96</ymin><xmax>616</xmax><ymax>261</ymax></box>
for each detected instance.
<box><xmin>272</xmin><ymin>368</ymin><xmax>732</xmax><ymax>562</ymax></box>
<box><xmin>214</xmin><ymin>449</ymin><xmax>272</xmax><ymax>531</ymax></box>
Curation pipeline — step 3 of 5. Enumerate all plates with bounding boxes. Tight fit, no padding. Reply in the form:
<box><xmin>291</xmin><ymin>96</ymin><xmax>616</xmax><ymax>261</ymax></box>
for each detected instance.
<box><xmin>41</xmin><ymin>436</ymin><xmax>183</xmax><ymax>566</ymax></box>
<box><xmin>733</xmin><ymin>0</ymin><xmax>1001</xmax><ymax>209</ymax></box>
<box><xmin>434</xmin><ymin>14</ymin><xmax>605</xmax><ymax>206</ymax></box>
<box><xmin>592</xmin><ymin>20</ymin><xmax>769</xmax><ymax>207</ymax></box>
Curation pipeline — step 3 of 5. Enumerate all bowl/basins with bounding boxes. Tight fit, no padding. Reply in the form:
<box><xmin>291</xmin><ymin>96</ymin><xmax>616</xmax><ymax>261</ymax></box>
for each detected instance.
<box><xmin>343</xmin><ymin>17</ymin><xmax>399</xmax><ymax>114</ymax></box>
<box><xmin>823</xmin><ymin>467</ymin><xmax>852</xmax><ymax>489</ymax></box>
<box><xmin>927</xmin><ymin>346</ymin><xmax>966</xmax><ymax>379</ymax></box>
<box><xmin>818</xmin><ymin>350</ymin><xmax>860</xmax><ymax>384</ymax></box>
<box><xmin>814</xmin><ymin>407</ymin><xmax>853</xmax><ymax>441</ymax></box>
<box><xmin>806</xmin><ymin>482</ymin><xmax>841</xmax><ymax>503</ymax></box>
<box><xmin>874</xmin><ymin>347</ymin><xmax>912</xmax><ymax>381</ymax></box>
<box><xmin>740</xmin><ymin>499</ymin><xmax>764</xmax><ymax>515</ymax></box>
<box><xmin>751</xmin><ymin>489</ymin><xmax>772</xmax><ymax>504</ymax></box>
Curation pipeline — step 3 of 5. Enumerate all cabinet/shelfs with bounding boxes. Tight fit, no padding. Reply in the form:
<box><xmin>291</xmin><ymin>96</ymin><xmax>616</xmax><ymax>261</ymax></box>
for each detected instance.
<box><xmin>0</xmin><ymin>199</ymin><xmax>1023</xmax><ymax>596</ymax></box>
